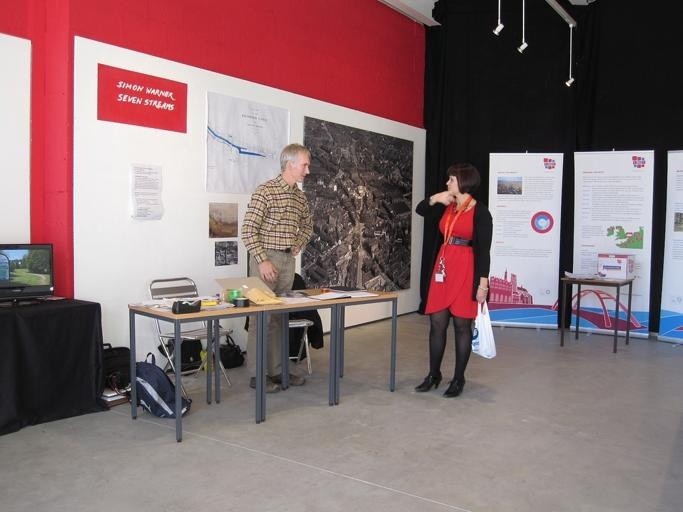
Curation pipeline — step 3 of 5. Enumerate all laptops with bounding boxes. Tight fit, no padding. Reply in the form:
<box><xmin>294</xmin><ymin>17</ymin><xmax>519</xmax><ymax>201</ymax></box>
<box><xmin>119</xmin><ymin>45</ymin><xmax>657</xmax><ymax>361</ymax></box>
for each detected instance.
<box><xmin>214</xmin><ymin>276</ymin><xmax>305</xmax><ymax>298</ymax></box>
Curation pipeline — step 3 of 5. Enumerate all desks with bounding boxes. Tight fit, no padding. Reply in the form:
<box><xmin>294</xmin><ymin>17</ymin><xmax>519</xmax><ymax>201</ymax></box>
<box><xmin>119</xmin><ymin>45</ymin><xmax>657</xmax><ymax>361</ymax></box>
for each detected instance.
<box><xmin>120</xmin><ymin>299</ymin><xmax>260</xmax><ymax>445</ymax></box>
<box><xmin>558</xmin><ymin>275</ymin><xmax>637</xmax><ymax>354</ymax></box>
<box><xmin>247</xmin><ymin>291</ymin><xmax>336</xmax><ymax>425</ymax></box>
<box><xmin>305</xmin><ymin>288</ymin><xmax>398</xmax><ymax>407</ymax></box>
<box><xmin>1</xmin><ymin>297</ymin><xmax>107</xmax><ymax>438</ymax></box>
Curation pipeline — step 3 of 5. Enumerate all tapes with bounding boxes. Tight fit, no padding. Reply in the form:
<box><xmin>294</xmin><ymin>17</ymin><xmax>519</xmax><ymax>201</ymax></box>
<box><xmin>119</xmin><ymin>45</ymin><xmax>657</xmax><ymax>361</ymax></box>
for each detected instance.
<box><xmin>182</xmin><ymin>301</ymin><xmax>191</xmax><ymax>305</ymax></box>
<box><xmin>233</xmin><ymin>298</ymin><xmax>249</xmax><ymax>307</ymax></box>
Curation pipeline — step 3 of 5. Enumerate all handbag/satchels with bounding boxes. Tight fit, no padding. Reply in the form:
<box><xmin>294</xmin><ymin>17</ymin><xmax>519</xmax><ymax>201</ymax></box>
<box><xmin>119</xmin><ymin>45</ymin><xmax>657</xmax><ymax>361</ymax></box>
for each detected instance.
<box><xmin>211</xmin><ymin>341</ymin><xmax>246</xmax><ymax>370</ymax></box>
<box><xmin>165</xmin><ymin>336</ymin><xmax>206</xmax><ymax>373</ymax></box>
<box><xmin>98</xmin><ymin>345</ymin><xmax>134</xmax><ymax>390</ymax></box>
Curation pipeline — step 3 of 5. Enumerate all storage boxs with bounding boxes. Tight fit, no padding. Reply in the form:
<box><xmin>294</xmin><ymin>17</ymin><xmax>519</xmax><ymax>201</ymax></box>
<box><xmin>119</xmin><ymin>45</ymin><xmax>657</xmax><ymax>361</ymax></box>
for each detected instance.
<box><xmin>596</xmin><ymin>252</ymin><xmax>636</xmax><ymax>279</ymax></box>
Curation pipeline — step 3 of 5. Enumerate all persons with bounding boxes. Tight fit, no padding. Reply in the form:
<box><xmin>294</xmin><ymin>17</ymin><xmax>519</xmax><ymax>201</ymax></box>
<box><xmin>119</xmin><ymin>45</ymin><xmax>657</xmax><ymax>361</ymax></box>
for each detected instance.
<box><xmin>413</xmin><ymin>160</ymin><xmax>492</xmax><ymax>397</ymax></box>
<box><xmin>241</xmin><ymin>144</ymin><xmax>313</xmax><ymax>393</ymax></box>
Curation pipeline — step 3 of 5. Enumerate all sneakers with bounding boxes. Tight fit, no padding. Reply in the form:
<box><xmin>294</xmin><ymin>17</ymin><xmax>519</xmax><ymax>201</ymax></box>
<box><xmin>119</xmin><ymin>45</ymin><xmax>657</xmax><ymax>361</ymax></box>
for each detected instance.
<box><xmin>249</xmin><ymin>376</ymin><xmax>280</xmax><ymax>394</ymax></box>
<box><xmin>267</xmin><ymin>371</ymin><xmax>306</xmax><ymax>386</ymax></box>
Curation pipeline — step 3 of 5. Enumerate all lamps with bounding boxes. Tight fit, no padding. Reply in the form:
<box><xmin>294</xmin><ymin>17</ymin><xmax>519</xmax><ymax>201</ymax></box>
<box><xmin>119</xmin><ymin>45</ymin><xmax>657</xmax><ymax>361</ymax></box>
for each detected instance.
<box><xmin>491</xmin><ymin>0</ymin><xmax>576</xmax><ymax>87</ymax></box>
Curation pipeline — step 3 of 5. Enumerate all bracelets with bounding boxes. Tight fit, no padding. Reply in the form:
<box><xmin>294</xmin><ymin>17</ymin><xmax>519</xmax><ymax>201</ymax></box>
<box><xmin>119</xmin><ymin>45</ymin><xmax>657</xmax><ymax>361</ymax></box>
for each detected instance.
<box><xmin>479</xmin><ymin>285</ymin><xmax>488</xmax><ymax>292</ymax></box>
<box><xmin>430</xmin><ymin>195</ymin><xmax>435</xmax><ymax>205</ymax></box>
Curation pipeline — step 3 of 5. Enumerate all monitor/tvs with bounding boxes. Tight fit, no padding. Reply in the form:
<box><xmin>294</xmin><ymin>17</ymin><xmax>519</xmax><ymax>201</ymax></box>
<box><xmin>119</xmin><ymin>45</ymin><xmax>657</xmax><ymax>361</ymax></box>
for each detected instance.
<box><xmin>0</xmin><ymin>244</ymin><xmax>54</xmax><ymax>308</ymax></box>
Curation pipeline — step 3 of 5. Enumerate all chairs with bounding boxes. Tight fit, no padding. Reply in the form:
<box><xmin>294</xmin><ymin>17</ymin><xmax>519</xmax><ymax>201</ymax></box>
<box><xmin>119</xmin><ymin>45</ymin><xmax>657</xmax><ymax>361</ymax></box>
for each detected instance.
<box><xmin>150</xmin><ymin>277</ymin><xmax>231</xmax><ymax>397</ymax></box>
<box><xmin>288</xmin><ymin>274</ymin><xmax>315</xmax><ymax>375</ymax></box>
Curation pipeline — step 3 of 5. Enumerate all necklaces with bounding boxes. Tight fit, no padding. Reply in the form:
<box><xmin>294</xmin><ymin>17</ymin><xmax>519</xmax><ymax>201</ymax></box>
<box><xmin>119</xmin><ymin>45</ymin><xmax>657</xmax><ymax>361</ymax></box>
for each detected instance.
<box><xmin>454</xmin><ymin>203</ymin><xmax>467</xmax><ymax>214</ymax></box>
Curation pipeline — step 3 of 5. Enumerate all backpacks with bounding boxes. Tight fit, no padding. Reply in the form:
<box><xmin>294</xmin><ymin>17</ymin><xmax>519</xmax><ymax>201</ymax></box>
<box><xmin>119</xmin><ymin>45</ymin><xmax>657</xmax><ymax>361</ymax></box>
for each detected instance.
<box><xmin>130</xmin><ymin>353</ymin><xmax>191</xmax><ymax>419</ymax></box>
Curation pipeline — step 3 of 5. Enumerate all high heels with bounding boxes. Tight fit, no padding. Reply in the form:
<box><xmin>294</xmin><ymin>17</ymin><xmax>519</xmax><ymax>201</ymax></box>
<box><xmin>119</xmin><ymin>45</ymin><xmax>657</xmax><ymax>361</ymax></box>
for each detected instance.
<box><xmin>414</xmin><ymin>372</ymin><xmax>442</xmax><ymax>393</ymax></box>
<box><xmin>442</xmin><ymin>376</ymin><xmax>465</xmax><ymax>397</ymax></box>
